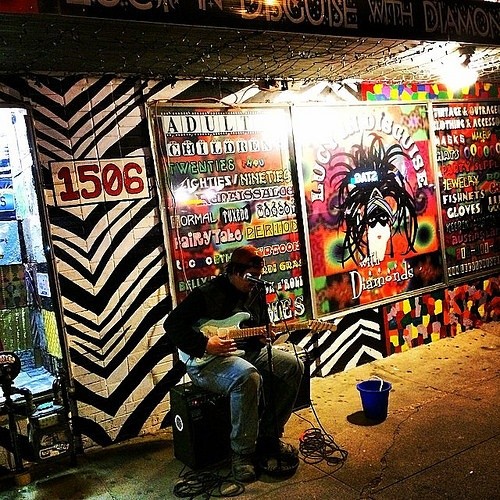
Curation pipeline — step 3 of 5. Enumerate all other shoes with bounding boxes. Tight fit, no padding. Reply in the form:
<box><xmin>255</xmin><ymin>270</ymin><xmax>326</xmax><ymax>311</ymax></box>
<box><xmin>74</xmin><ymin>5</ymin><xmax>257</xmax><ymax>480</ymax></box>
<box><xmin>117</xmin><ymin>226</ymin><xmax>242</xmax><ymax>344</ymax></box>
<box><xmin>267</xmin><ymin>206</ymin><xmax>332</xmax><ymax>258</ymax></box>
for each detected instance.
<box><xmin>275</xmin><ymin>439</ymin><xmax>299</xmax><ymax>456</ymax></box>
<box><xmin>231</xmin><ymin>452</ymin><xmax>257</xmax><ymax>482</ymax></box>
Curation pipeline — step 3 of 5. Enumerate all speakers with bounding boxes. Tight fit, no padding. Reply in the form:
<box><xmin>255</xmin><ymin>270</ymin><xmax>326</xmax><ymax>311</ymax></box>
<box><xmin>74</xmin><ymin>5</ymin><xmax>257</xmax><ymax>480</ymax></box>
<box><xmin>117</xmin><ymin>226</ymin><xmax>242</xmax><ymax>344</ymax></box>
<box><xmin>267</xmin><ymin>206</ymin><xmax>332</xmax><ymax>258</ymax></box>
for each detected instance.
<box><xmin>170</xmin><ymin>381</ymin><xmax>232</xmax><ymax>473</ymax></box>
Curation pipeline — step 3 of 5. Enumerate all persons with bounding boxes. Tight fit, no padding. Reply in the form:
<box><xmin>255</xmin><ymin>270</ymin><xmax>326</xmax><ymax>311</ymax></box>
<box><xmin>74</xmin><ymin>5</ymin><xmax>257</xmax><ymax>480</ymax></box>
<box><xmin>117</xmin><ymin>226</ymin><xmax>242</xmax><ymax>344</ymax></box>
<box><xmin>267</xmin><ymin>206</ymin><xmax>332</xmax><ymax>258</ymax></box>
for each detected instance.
<box><xmin>164</xmin><ymin>244</ymin><xmax>302</xmax><ymax>481</ymax></box>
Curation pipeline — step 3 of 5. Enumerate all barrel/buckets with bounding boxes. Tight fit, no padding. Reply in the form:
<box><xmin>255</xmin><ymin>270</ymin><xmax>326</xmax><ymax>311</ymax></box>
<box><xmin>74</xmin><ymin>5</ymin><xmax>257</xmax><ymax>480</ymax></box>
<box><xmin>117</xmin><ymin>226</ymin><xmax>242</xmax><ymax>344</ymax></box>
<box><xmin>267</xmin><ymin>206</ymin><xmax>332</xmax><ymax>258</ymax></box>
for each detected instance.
<box><xmin>356</xmin><ymin>379</ymin><xmax>391</xmax><ymax>419</ymax></box>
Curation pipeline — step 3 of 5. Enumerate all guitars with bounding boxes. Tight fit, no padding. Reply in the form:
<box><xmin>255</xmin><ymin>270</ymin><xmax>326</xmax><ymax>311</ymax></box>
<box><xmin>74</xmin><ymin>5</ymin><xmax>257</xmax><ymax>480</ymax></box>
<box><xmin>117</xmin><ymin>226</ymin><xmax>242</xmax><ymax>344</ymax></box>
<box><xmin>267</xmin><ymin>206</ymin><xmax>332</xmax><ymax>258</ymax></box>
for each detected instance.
<box><xmin>177</xmin><ymin>310</ymin><xmax>339</xmax><ymax>367</ymax></box>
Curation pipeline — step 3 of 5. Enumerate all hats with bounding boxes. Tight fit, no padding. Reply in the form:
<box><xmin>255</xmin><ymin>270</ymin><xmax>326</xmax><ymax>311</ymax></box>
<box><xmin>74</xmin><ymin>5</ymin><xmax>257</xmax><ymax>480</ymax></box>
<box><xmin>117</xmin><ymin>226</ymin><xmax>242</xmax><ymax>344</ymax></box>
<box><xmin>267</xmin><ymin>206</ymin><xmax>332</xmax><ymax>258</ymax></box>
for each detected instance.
<box><xmin>225</xmin><ymin>246</ymin><xmax>266</xmax><ymax>269</ymax></box>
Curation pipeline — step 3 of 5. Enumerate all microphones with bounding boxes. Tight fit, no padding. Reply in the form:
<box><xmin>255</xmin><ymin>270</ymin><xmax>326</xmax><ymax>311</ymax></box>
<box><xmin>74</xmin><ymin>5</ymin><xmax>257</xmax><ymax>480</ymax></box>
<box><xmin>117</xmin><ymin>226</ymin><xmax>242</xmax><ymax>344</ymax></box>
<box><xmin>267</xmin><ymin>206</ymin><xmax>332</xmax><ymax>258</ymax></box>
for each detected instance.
<box><xmin>242</xmin><ymin>273</ymin><xmax>271</xmax><ymax>287</ymax></box>
